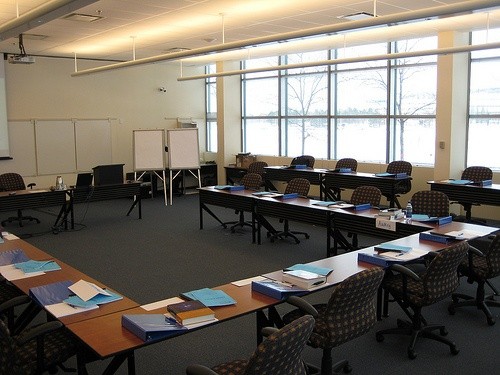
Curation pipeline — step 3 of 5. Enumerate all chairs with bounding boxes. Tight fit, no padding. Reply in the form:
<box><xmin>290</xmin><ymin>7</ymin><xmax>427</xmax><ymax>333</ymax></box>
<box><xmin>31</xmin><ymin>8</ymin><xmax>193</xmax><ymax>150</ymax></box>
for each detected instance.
<box><xmin>330</xmin><ymin>186</ymin><xmax>382</xmax><ymax>253</ymax></box>
<box><xmin>267</xmin><ymin>179</ymin><xmax>311</xmax><ymax>244</ymax></box>
<box><xmin>447</xmin><ymin>231</ymin><xmax>499</xmax><ymax>325</ymax></box>
<box><xmin>380</xmin><ymin>161</ymin><xmax>412</xmax><ymax>208</ymax></box>
<box><xmin>334</xmin><ymin>158</ymin><xmax>357</xmax><ymax>200</ymax></box>
<box><xmin>222</xmin><ymin>174</ymin><xmax>261</xmax><ymax>233</ymax></box>
<box><xmin>411</xmin><ymin>191</ymin><xmax>450</xmax><ymax>218</ymax></box>
<box><xmin>292</xmin><ymin>156</ymin><xmax>314</xmax><ymax>168</ymax></box>
<box><xmin>186</xmin><ymin>314</ymin><xmax>315</xmax><ymax>375</ymax></box>
<box><xmin>377</xmin><ymin>240</ymin><xmax>469</xmax><ymax>359</ymax></box>
<box><xmin>249</xmin><ymin>162</ymin><xmax>268</xmax><ymax>186</ymax></box>
<box><xmin>456</xmin><ymin>166</ymin><xmax>491</xmax><ymax>224</ymax></box>
<box><xmin>270</xmin><ymin>267</ymin><xmax>384</xmax><ymax>374</ymax></box>
<box><xmin>0</xmin><ymin>295</ymin><xmax>76</xmax><ymax>375</ymax></box>
<box><xmin>0</xmin><ymin>173</ymin><xmax>40</xmax><ymax>227</ymax></box>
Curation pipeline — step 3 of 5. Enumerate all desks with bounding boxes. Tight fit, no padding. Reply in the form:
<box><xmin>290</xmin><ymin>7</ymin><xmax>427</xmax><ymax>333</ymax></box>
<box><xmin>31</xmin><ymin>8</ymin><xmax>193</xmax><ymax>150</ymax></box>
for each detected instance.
<box><xmin>0</xmin><ymin>165</ymin><xmax>500</xmax><ymax>375</ymax></box>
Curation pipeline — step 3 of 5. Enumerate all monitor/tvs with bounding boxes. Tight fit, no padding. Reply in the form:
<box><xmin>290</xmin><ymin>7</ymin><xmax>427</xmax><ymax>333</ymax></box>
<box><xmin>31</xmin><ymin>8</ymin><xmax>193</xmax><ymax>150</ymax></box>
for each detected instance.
<box><xmin>76</xmin><ymin>172</ymin><xmax>94</xmax><ymax>188</ymax></box>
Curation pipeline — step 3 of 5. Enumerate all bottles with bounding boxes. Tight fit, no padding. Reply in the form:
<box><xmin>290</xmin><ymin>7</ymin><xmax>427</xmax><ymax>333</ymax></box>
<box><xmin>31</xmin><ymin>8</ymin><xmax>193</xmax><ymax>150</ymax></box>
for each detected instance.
<box><xmin>406</xmin><ymin>201</ymin><xmax>413</xmax><ymax>223</ymax></box>
<box><xmin>56</xmin><ymin>176</ymin><xmax>63</xmax><ymax>189</ymax></box>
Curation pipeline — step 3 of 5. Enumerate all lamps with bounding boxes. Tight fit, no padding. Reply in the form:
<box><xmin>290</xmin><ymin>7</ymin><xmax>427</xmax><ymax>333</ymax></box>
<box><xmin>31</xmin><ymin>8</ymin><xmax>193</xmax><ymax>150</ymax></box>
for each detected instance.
<box><xmin>0</xmin><ymin>0</ymin><xmax>499</xmax><ymax>81</ymax></box>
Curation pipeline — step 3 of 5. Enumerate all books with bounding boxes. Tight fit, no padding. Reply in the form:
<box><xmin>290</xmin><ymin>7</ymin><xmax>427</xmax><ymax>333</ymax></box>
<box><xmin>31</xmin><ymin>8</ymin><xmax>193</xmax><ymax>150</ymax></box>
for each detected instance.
<box><xmin>282</xmin><ymin>269</ymin><xmax>326</xmax><ymax>290</ymax></box>
<box><xmin>167</xmin><ymin>300</ymin><xmax>215</xmax><ymax>325</ymax></box>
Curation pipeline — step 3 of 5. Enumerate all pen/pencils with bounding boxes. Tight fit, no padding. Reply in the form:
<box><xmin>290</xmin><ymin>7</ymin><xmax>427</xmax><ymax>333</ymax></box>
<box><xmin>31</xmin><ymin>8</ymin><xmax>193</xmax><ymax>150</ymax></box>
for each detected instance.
<box><xmin>68</xmin><ymin>304</ymin><xmax>76</xmax><ymax>308</ymax></box>
<box><xmin>459</xmin><ymin>232</ymin><xmax>464</xmax><ymax>236</ymax></box>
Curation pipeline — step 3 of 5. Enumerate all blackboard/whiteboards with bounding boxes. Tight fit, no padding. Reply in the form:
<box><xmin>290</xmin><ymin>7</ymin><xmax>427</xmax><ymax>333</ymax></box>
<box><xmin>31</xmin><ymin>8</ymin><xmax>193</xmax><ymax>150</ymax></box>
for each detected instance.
<box><xmin>166</xmin><ymin>128</ymin><xmax>201</xmax><ymax>169</ymax></box>
<box><xmin>132</xmin><ymin>129</ymin><xmax>166</xmax><ymax>172</ymax></box>
<box><xmin>0</xmin><ymin>117</ymin><xmax>36</xmax><ymax>177</ymax></box>
<box><xmin>75</xmin><ymin>117</ymin><xmax>113</xmax><ymax>173</ymax></box>
<box><xmin>34</xmin><ymin>117</ymin><xmax>77</xmax><ymax>176</ymax></box>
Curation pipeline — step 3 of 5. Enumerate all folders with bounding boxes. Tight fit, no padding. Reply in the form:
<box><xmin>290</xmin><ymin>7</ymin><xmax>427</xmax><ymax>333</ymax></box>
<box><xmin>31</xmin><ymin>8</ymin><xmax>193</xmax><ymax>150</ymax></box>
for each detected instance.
<box><xmin>271</xmin><ymin>193</ymin><xmax>298</xmax><ymax>199</ymax></box>
<box><xmin>469</xmin><ymin>180</ymin><xmax>492</xmax><ymax>186</ymax></box>
<box><xmin>357</xmin><ymin>252</ymin><xmax>402</xmax><ymax>266</ymax></box>
<box><xmin>326</xmin><ymin>168</ymin><xmax>352</xmax><ymax>174</ymax></box>
<box><xmin>386</xmin><ymin>172</ymin><xmax>407</xmax><ymax>177</ymax></box>
<box><xmin>29</xmin><ymin>280</ymin><xmax>76</xmax><ymax>307</ymax></box>
<box><xmin>284</xmin><ymin>165</ymin><xmax>307</xmax><ymax>168</ymax></box>
<box><xmin>251</xmin><ymin>281</ymin><xmax>310</xmax><ymax>300</ymax></box>
<box><xmin>418</xmin><ymin>216</ymin><xmax>453</xmax><ymax>225</ymax></box>
<box><xmin>121</xmin><ymin>314</ymin><xmax>187</xmax><ymax>342</ymax></box>
<box><xmin>340</xmin><ymin>203</ymin><xmax>371</xmax><ymax>210</ymax></box>
<box><xmin>218</xmin><ymin>185</ymin><xmax>246</xmax><ymax>191</ymax></box>
<box><xmin>419</xmin><ymin>232</ymin><xmax>456</xmax><ymax>245</ymax></box>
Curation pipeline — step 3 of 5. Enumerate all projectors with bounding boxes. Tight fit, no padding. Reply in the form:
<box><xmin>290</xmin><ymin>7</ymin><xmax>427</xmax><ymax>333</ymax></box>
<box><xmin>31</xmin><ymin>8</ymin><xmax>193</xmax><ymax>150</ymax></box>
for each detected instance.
<box><xmin>7</xmin><ymin>54</ymin><xmax>36</xmax><ymax>64</ymax></box>
